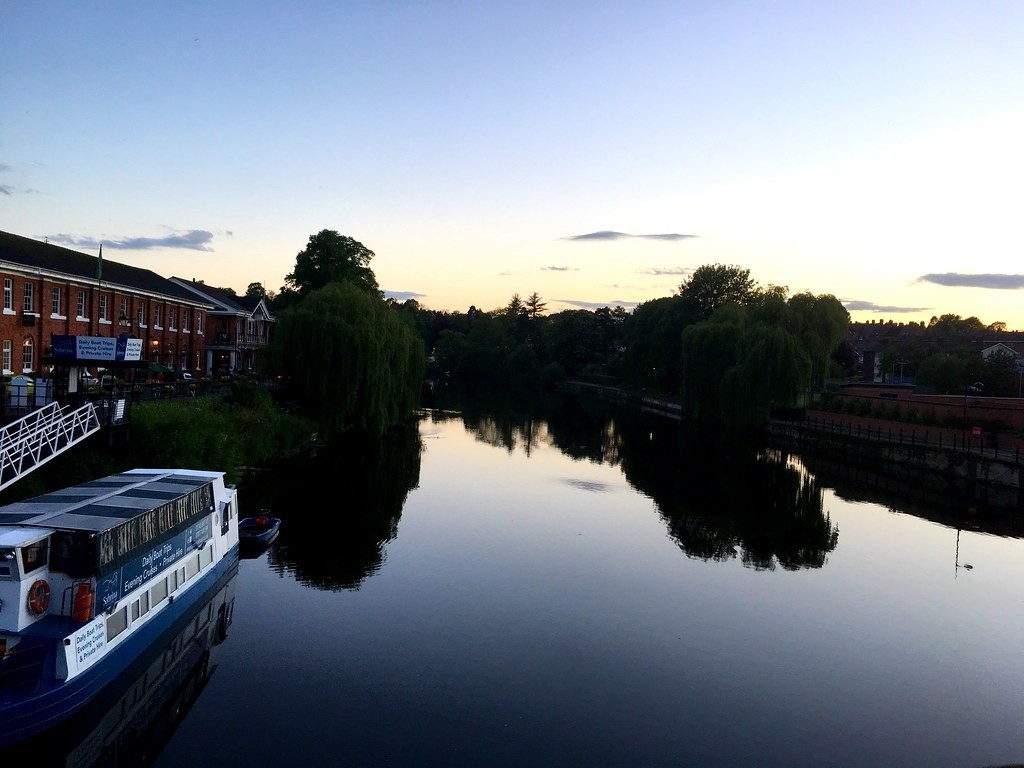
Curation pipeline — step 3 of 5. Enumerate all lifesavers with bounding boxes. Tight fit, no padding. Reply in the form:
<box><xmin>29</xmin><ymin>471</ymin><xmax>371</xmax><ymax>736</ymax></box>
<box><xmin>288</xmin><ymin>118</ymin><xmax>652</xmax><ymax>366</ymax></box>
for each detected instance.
<box><xmin>28</xmin><ymin>580</ymin><xmax>52</xmax><ymax>614</ymax></box>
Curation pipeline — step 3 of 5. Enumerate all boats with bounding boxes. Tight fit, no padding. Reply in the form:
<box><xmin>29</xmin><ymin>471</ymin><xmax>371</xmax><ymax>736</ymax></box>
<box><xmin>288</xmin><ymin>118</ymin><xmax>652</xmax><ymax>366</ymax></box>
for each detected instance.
<box><xmin>0</xmin><ymin>558</ymin><xmax>242</xmax><ymax>767</ymax></box>
<box><xmin>0</xmin><ymin>465</ymin><xmax>241</xmax><ymax>748</ymax></box>
<box><xmin>237</xmin><ymin>506</ymin><xmax>282</xmax><ymax>548</ymax></box>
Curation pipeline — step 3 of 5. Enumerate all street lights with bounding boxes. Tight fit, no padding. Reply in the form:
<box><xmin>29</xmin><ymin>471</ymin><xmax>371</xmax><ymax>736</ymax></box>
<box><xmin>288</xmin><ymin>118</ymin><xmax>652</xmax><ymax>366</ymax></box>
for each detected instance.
<box><xmin>896</xmin><ymin>359</ymin><xmax>910</xmax><ymax>384</ymax></box>
